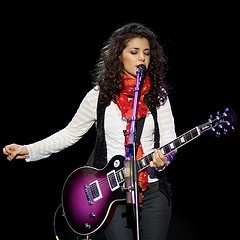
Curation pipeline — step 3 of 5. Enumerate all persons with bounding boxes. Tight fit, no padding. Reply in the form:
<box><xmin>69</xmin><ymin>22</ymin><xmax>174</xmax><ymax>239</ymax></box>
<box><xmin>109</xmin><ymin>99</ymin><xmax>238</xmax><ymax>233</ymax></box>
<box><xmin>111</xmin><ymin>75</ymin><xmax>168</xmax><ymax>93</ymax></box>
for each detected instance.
<box><xmin>3</xmin><ymin>23</ymin><xmax>178</xmax><ymax>240</ymax></box>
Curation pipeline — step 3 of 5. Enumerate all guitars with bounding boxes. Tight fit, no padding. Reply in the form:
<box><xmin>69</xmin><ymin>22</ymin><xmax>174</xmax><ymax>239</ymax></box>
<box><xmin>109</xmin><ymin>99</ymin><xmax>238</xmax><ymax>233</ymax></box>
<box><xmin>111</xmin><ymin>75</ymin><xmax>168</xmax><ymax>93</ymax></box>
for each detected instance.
<box><xmin>61</xmin><ymin>108</ymin><xmax>237</xmax><ymax>236</ymax></box>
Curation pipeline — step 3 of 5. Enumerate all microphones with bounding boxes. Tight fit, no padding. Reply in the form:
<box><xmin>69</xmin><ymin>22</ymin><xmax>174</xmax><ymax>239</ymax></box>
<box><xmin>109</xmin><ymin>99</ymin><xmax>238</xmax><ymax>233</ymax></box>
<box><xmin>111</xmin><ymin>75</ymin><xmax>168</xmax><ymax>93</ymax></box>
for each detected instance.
<box><xmin>137</xmin><ymin>64</ymin><xmax>146</xmax><ymax>90</ymax></box>
